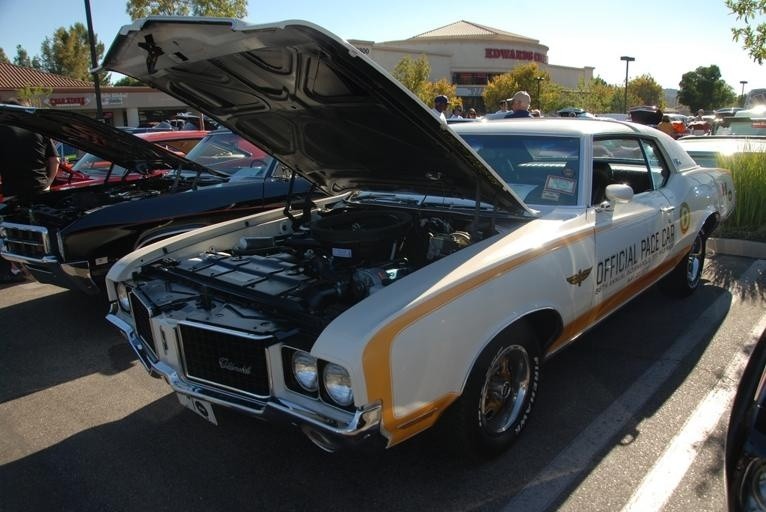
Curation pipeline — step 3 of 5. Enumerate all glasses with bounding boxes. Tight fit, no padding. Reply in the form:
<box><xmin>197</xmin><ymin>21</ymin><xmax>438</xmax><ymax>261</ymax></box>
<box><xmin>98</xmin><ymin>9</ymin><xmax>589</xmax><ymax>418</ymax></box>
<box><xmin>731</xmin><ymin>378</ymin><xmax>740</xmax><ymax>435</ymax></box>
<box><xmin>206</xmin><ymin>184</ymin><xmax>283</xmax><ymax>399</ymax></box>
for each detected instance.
<box><xmin>456</xmin><ymin>108</ymin><xmax>461</xmax><ymax>112</ymax></box>
<box><xmin>469</xmin><ymin>113</ymin><xmax>476</xmax><ymax>115</ymax></box>
<box><xmin>531</xmin><ymin>110</ymin><xmax>539</xmax><ymax>114</ymax></box>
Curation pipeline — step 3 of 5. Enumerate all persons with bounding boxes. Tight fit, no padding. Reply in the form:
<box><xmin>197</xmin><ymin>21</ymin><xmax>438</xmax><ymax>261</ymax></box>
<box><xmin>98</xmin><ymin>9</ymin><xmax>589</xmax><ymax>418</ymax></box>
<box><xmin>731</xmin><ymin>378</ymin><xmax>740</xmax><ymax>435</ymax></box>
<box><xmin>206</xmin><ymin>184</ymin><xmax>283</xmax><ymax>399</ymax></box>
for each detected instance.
<box><xmin>0</xmin><ymin>95</ymin><xmax>61</xmax><ymax>200</ymax></box>
<box><xmin>503</xmin><ymin>90</ymin><xmax>534</xmax><ymax>118</ymax></box>
<box><xmin>693</xmin><ymin>108</ymin><xmax>705</xmax><ymax>122</ymax></box>
<box><xmin>657</xmin><ymin>116</ymin><xmax>674</xmax><ymax>138</ymax></box>
<box><xmin>449</xmin><ymin>105</ymin><xmax>464</xmax><ymax>119</ymax></box>
<box><xmin>530</xmin><ymin>109</ymin><xmax>541</xmax><ymax>118</ymax></box>
<box><xmin>430</xmin><ymin>95</ymin><xmax>448</xmax><ymax>126</ymax></box>
<box><xmin>496</xmin><ymin>100</ymin><xmax>510</xmax><ymax>114</ymax></box>
<box><xmin>466</xmin><ymin>108</ymin><xmax>477</xmax><ymax>118</ymax></box>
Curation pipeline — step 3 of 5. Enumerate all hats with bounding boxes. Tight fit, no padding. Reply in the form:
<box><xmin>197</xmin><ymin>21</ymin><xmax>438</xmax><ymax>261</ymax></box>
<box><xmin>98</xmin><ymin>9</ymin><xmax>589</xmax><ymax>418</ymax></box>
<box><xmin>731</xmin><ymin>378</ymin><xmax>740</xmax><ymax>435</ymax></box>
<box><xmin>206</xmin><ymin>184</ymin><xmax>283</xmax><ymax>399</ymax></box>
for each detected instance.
<box><xmin>435</xmin><ymin>96</ymin><xmax>452</xmax><ymax>103</ymax></box>
<box><xmin>507</xmin><ymin>91</ymin><xmax>531</xmax><ymax>103</ymax></box>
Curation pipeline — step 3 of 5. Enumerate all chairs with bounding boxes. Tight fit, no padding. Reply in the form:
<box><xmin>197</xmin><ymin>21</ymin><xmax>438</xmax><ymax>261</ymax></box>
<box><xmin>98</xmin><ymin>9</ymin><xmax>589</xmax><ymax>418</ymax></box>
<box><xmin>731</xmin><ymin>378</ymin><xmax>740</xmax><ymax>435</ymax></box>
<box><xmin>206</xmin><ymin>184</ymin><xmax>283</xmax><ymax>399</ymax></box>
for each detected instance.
<box><xmin>476</xmin><ymin>146</ymin><xmax>616</xmax><ymax>210</ymax></box>
